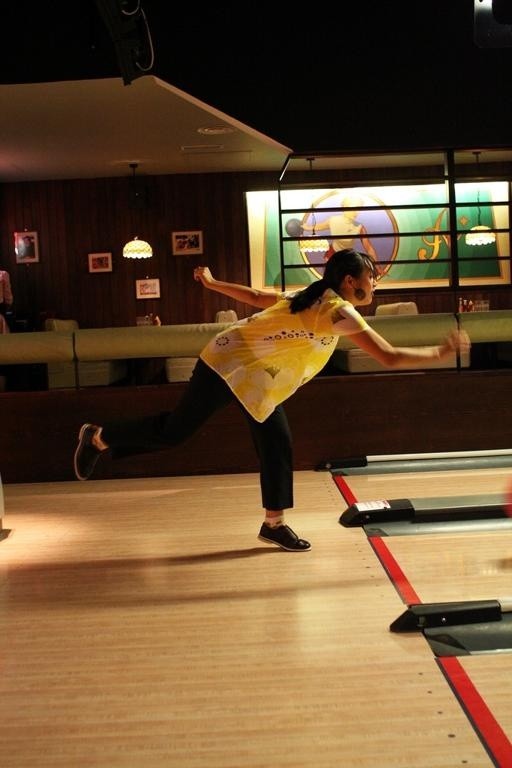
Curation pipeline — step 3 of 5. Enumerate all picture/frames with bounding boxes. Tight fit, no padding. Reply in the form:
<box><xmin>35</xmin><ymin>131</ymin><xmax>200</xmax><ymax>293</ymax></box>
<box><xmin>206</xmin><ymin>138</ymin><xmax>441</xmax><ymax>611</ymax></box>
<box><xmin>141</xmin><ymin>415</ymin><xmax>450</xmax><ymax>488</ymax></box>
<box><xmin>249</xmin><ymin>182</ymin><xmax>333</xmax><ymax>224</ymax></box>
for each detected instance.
<box><xmin>170</xmin><ymin>229</ymin><xmax>204</xmax><ymax>257</ymax></box>
<box><xmin>14</xmin><ymin>230</ymin><xmax>40</xmax><ymax>264</ymax></box>
<box><xmin>88</xmin><ymin>252</ymin><xmax>113</xmax><ymax>273</ymax></box>
<box><xmin>133</xmin><ymin>276</ymin><xmax>163</xmax><ymax>302</ymax></box>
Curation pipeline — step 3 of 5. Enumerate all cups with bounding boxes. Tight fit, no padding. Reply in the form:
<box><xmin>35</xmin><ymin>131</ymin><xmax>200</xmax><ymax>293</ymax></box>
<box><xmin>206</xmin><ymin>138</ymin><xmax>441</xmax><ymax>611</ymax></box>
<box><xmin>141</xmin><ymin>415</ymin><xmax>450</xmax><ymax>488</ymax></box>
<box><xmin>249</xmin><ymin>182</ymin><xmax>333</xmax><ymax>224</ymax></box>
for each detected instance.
<box><xmin>136</xmin><ymin>317</ymin><xmax>145</xmax><ymax>326</ymax></box>
<box><xmin>473</xmin><ymin>300</ymin><xmax>490</xmax><ymax>312</ymax></box>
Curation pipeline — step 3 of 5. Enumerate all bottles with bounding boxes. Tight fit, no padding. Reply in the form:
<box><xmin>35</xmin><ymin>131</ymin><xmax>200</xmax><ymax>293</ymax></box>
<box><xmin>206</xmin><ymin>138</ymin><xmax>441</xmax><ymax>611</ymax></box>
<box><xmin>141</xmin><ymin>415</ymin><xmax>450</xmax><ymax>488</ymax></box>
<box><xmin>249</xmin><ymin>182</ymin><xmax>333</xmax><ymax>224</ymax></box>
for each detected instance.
<box><xmin>458</xmin><ymin>298</ymin><xmax>473</xmax><ymax>313</ymax></box>
<box><xmin>145</xmin><ymin>313</ymin><xmax>161</xmax><ymax>326</ymax></box>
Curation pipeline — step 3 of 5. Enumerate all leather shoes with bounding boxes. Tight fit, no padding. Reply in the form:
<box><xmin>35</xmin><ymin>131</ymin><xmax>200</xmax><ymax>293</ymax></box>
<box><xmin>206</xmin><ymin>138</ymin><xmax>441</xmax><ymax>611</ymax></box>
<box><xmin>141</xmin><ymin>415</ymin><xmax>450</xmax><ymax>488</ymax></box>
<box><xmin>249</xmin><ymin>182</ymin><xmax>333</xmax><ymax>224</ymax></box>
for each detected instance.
<box><xmin>257</xmin><ymin>522</ymin><xmax>312</xmax><ymax>552</ymax></box>
<box><xmin>74</xmin><ymin>424</ymin><xmax>101</xmax><ymax>481</ymax></box>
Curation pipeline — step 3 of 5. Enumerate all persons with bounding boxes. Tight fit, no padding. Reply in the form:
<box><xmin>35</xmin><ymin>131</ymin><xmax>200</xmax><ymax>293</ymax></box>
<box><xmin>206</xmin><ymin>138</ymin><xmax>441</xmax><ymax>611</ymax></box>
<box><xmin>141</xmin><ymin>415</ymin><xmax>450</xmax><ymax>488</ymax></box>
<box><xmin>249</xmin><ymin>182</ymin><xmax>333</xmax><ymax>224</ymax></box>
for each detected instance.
<box><xmin>300</xmin><ymin>197</ymin><xmax>385</xmax><ymax>274</ymax></box>
<box><xmin>75</xmin><ymin>249</ymin><xmax>472</xmax><ymax>552</ymax></box>
<box><xmin>20</xmin><ymin>236</ymin><xmax>35</xmax><ymax>259</ymax></box>
<box><xmin>0</xmin><ymin>271</ymin><xmax>13</xmax><ymax>333</ymax></box>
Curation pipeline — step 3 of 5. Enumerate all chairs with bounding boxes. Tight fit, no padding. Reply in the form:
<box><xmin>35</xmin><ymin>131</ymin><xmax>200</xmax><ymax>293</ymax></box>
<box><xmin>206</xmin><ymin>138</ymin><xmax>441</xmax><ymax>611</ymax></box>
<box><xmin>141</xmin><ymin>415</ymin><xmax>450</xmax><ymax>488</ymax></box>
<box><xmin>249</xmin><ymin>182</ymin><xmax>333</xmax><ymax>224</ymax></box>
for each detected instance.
<box><xmin>164</xmin><ymin>308</ymin><xmax>241</xmax><ymax>383</ymax></box>
<box><xmin>47</xmin><ymin>319</ymin><xmax>121</xmax><ymax>386</ymax></box>
<box><xmin>334</xmin><ymin>301</ymin><xmax>406</xmax><ymax>374</ymax></box>
<box><xmin>405</xmin><ymin>302</ymin><xmax>472</xmax><ymax>369</ymax></box>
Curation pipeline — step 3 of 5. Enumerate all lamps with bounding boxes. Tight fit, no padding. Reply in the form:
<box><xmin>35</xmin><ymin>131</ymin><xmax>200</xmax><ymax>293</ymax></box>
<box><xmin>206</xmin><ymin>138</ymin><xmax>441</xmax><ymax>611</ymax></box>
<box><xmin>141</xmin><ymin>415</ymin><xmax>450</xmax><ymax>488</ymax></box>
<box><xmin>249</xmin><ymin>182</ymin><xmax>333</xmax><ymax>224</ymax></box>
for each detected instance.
<box><xmin>298</xmin><ymin>159</ymin><xmax>331</xmax><ymax>253</ymax></box>
<box><xmin>122</xmin><ymin>163</ymin><xmax>155</xmax><ymax>258</ymax></box>
<box><xmin>465</xmin><ymin>151</ymin><xmax>497</xmax><ymax>247</ymax></box>
